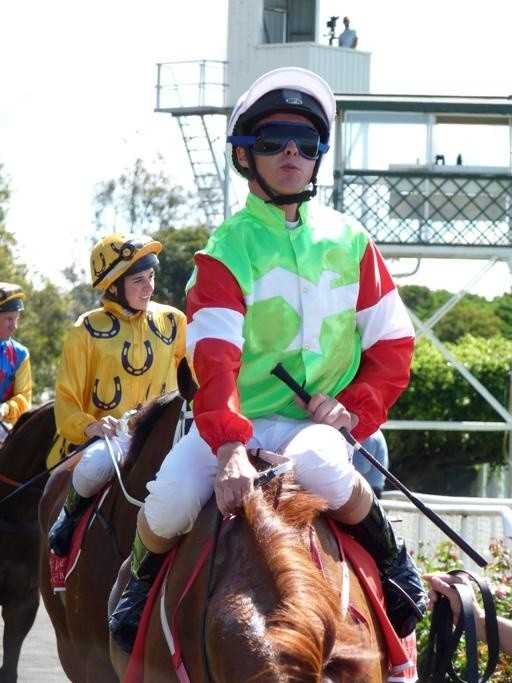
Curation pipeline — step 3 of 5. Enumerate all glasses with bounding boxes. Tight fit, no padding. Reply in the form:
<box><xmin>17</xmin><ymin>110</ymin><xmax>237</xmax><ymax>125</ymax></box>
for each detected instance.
<box><xmin>249</xmin><ymin>119</ymin><xmax>322</xmax><ymax>160</ymax></box>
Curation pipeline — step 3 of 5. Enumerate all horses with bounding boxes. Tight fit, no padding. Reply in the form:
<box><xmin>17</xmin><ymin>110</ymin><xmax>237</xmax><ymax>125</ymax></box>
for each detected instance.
<box><xmin>106</xmin><ymin>467</ymin><xmax>400</xmax><ymax>682</ymax></box>
<box><xmin>32</xmin><ymin>355</ymin><xmax>201</xmax><ymax>683</ymax></box>
<box><xmin>1</xmin><ymin>396</ymin><xmax>58</xmax><ymax>683</ymax></box>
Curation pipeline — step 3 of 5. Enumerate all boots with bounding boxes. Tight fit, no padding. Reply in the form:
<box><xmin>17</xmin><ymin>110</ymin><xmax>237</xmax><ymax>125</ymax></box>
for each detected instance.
<box><xmin>332</xmin><ymin>489</ymin><xmax>427</xmax><ymax>638</ymax></box>
<box><xmin>46</xmin><ymin>482</ymin><xmax>98</xmax><ymax>557</ymax></box>
<box><xmin>108</xmin><ymin>529</ymin><xmax>173</xmax><ymax>653</ymax></box>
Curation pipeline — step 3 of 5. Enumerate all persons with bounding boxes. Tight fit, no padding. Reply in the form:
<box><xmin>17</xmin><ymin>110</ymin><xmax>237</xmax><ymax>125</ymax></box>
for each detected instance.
<box><xmin>0</xmin><ymin>282</ymin><xmax>33</xmax><ymax>448</ymax></box>
<box><xmin>47</xmin><ymin>234</ymin><xmax>194</xmax><ymax>556</ymax></box>
<box><xmin>337</xmin><ymin>16</ymin><xmax>358</xmax><ymax>48</ymax></box>
<box><xmin>108</xmin><ymin>63</ymin><xmax>429</xmax><ymax>657</ymax></box>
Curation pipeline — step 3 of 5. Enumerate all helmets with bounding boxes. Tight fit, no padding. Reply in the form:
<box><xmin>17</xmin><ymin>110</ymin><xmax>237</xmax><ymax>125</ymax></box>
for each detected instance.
<box><xmin>226</xmin><ymin>66</ymin><xmax>337</xmax><ymax>179</ymax></box>
<box><xmin>0</xmin><ymin>282</ymin><xmax>25</xmax><ymax>312</ymax></box>
<box><xmin>90</xmin><ymin>232</ymin><xmax>163</xmax><ymax>290</ymax></box>
